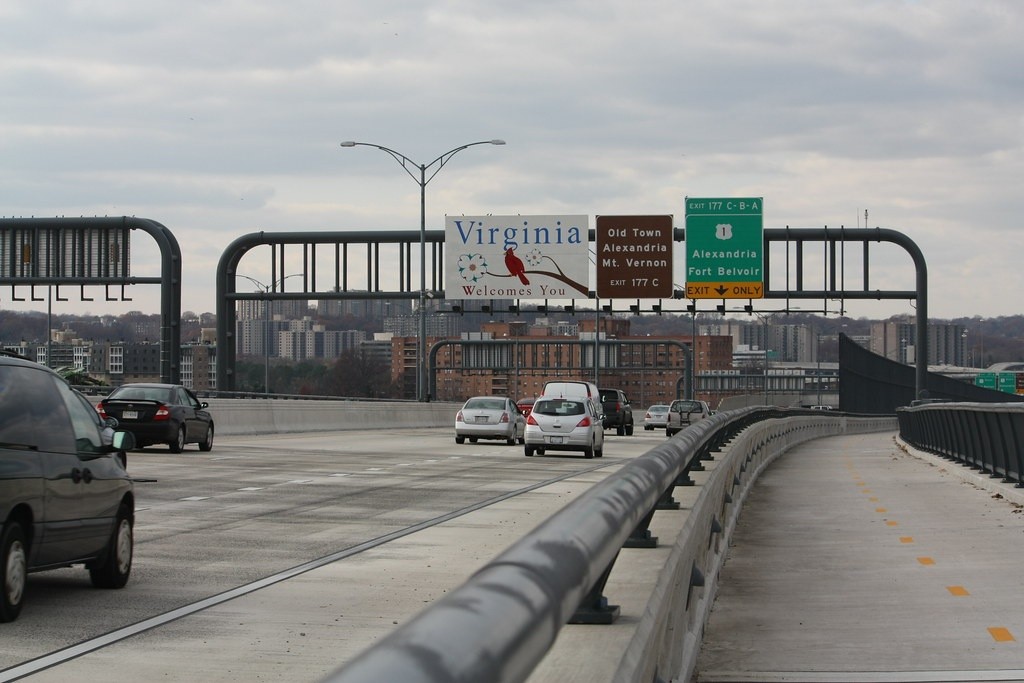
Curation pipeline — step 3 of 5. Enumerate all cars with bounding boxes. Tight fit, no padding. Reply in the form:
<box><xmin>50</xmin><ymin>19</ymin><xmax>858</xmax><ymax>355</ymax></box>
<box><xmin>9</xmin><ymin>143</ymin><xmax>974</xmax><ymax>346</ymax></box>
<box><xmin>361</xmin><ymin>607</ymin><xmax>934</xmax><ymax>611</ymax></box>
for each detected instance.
<box><xmin>96</xmin><ymin>383</ymin><xmax>214</xmax><ymax>454</ymax></box>
<box><xmin>455</xmin><ymin>396</ymin><xmax>528</xmax><ymax>446</ymax></box>
<box><xmin>523</xmin><ymin>394</ymin><xmax>608</xmax><ymax>456</ymax></box>
<box><xmin>666</xmin><ymin>400</ymin><xmax>714</xmax><ymax>435</ymax></box>
<box><xmin>517</xmin><ymin>397</ymin><xmax>542</xmax><ymax>419</ymax></box>
<box><xmin>644</xmin><ymin>405</ymin><xmax>670</xmax><ymax>431</ymax></box>
<box><xmin>0</xmin><ymin>357</ymin><xmax>135</xmax><ymax>621</ymax></box>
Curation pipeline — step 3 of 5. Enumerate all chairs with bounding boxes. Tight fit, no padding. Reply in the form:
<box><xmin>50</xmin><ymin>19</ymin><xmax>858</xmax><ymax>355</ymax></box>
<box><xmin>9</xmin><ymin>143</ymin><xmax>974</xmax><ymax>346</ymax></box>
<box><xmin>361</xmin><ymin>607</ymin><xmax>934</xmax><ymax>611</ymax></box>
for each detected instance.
<box><xmin>543</xmin><ymin>407</ymin><xmax>556</xmax><ymax>413</ymax></box>
<box><xmin>567</xmin><ymin>408</ymin><xmax>579</xmax><ymax>413</ymax></box>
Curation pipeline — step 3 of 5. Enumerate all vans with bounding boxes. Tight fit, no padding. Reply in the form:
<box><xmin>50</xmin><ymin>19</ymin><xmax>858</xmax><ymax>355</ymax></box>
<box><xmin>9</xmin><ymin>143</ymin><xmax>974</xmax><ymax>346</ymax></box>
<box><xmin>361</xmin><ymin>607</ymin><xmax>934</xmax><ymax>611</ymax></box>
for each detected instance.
<box><xmin>542</xmin><ymin>381</ymin><xmax>606</xmax><ymax>425</ymax></box>
<box><xmin>811</xmin><ymin>405</ymin><xmax>833</xmax><ymax>410</ymax></box>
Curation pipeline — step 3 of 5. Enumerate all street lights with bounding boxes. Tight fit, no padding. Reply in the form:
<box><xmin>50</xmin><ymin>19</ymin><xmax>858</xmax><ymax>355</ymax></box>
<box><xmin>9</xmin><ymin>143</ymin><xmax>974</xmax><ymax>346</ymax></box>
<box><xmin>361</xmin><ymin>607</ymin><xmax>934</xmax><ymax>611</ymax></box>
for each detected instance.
<box><xmin>733</xmin><ymin>305</ymin><xmax>800</xmax><ymax>406</ymax></box>
<box><xmin>341</xmin><ymin>137</ymin><xmax>507</xmax><ymax>403</ymax></box>
<box><xmin>236</xmin><ymin>273</ymin><xmax>303</xmax><ymax>399</ymax></box>
<box><xmin>817</xmin><ymin>325</ymin><xmax>849</xmax><ymax>406</ymax></box>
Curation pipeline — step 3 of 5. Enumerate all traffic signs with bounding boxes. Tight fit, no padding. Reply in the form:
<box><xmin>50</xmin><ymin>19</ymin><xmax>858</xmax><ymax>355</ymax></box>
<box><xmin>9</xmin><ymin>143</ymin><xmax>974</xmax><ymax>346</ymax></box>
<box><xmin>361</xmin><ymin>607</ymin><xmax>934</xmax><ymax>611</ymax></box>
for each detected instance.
<box><xmin>685</xmin><ymin>196</ymin><xmax>764</xmax><ymax>300</ymax></box>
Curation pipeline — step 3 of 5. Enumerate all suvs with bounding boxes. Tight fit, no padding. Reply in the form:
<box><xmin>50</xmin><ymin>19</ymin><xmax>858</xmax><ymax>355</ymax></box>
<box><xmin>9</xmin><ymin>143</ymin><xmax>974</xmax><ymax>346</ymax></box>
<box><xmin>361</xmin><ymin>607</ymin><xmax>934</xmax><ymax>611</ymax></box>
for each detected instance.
<box><xmin>598</xmin><ymin>388</ymin><xmax>635</xmax><ymax>436</ymax></box>
